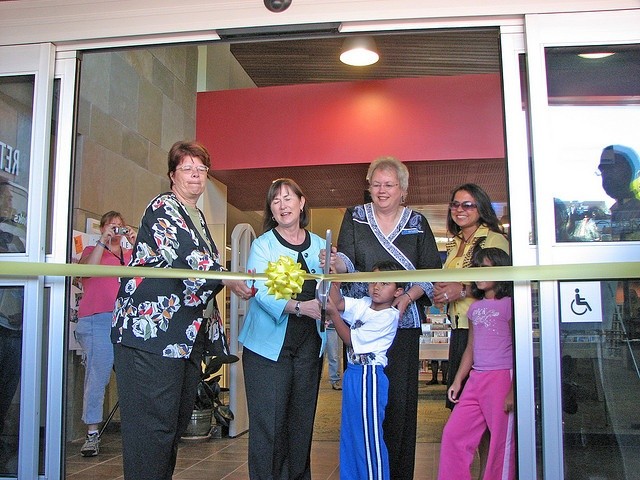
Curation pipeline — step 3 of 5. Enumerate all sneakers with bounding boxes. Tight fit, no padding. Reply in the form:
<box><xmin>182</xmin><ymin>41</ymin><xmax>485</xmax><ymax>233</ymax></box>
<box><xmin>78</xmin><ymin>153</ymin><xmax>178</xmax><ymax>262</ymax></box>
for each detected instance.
<box><xmin>81</xmin><ymin>432</ymin><xmax>102</xmax><ymax>457</ymax></box>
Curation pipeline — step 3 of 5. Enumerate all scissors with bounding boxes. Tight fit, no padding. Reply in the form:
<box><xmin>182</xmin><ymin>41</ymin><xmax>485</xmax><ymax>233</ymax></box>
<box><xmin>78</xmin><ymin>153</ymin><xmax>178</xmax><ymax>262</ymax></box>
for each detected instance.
<box><xmin>316</xmin><ymin>229</ymin><xmax>332</xmax><ymax>332</ymax></box>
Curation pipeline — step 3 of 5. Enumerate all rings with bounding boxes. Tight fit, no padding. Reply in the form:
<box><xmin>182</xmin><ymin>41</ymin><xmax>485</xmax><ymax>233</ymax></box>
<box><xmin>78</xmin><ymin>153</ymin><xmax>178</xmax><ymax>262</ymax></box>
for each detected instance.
<box><xmin>444</xmin><ymin>293</ymin><xmax>446</xmax><ymax>297</ymax></box>
<box><xmin>444</xmin><ymin>296</ymin><xmax>448</xmax><ymax>300</ymax></box>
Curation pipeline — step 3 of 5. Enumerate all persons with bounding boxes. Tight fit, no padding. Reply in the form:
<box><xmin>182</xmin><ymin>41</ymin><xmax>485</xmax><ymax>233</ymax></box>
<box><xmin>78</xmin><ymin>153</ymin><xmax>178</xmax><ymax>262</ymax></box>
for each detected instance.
<box><xmin>74</xmin><ymin>277</ymin><xmax>79</xmax><ymax>287</ymax></box>
<box><xmin>318</xmin><ymin>157</ymin><xmax>444</xmax><ymax>480</ymax></box>
<box><xmin>325</xmin><ymin>314</ymin><xmax>344</xmax><ymax>389</ymax></box>
<box><xmin>426</xmin><ymin>301</ymin><xmax>449</xmax><ymax>386</ymax></box>
<box><xmin>438</xmin><ymin>248</ymin><xmax>519</xmax><ymax>480</ymax></box>
<box><xmin>325</xmin><ymin>260</ymin><xmax>405</xmax><ymax>480</ymax></box>
<box><xmin>432</xmin><ymin>183</ymin><xmax>510</xmax><ymax>479</ymax></box>
<box><xmin>555</xmin><ymin>198</ymin><xmax>585</xmax><ymax>242</ymax></box>
<box><xmin>236</xmin><ymin>178</ymin><xmax>331</xmax><ymax>480</ymax></box>
<box><xmin>630</xmin><ymin>289</ymin><xmax>640</xmax><ymax>319</ymax></box>
<box><xmin>77</xmin><ymin>211</ymin><xmax>142</xmax><ymax>458</ymax></box>
<box><xmin>0</xmin><ymin>177</ymin><xmax>26</xmax><ymax>475</ymax></box>
<box><xmin>111</xmin><ymin>140</ymin><xmax>259</xmax><ymax>479</ymax></box>
<box><xmin>599</xmin><ymin>145</ymin><xmax>640</xmax><ymax>240</ymax></box>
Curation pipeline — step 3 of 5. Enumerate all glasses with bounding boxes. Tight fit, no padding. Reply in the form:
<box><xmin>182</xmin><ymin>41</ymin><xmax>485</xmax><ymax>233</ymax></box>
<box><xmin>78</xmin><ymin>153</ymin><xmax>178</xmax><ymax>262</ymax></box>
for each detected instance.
<box><xmin>176</xmin><ymin>165</ymin><xmax>209</xmax><ymax>174</ymax></box>
<box><xmin>449</xmin><ymin>200</ymin><xmax>477</xmax><ymax>210</ymax></box>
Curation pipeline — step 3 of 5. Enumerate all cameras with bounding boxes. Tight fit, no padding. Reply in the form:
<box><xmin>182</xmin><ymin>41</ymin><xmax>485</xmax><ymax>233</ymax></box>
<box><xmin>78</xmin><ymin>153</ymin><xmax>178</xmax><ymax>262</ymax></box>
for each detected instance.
<box><xmin>112</xmin><ymin>228</ymin><xmax>128</xmax><ymax>234</ymax></box>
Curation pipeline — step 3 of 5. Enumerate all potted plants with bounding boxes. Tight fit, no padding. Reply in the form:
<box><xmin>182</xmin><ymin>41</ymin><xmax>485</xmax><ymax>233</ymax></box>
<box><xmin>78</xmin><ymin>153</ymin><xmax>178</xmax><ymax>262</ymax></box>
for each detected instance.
<box><xmin>179</xmin><ymin>354</ymin><xmax>241</xmax><ymax>443</ymax></box>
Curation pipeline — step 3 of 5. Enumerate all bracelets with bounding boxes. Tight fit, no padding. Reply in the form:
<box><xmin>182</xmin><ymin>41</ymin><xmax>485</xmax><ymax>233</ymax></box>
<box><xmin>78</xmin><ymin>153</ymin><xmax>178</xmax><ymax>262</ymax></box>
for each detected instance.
<box><xmin>405</xmin><ymin>292</ymin><xmax>414</xmax><ymax>303</ymax></box>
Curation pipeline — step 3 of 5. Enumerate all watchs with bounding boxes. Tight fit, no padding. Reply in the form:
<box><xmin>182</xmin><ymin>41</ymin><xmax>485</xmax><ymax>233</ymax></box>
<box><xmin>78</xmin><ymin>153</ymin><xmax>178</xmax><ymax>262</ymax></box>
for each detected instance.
<box><xmin>295</xmin><ymin>301</ymin><xmax>300</xmax><ymax>317</ymax></box>
<box><xmin>459</xmin><ymin>284</ymin><xmax>466</xmax><ymax>299</ymax></box>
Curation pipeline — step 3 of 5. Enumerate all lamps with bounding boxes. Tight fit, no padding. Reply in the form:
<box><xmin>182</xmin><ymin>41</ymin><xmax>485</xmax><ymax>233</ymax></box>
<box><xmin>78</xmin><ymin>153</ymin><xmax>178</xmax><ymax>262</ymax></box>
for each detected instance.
<box><xmin>338</xmin><ymin>36</ymin><xmax>381</xmax><ymax>68</ymax></box>
